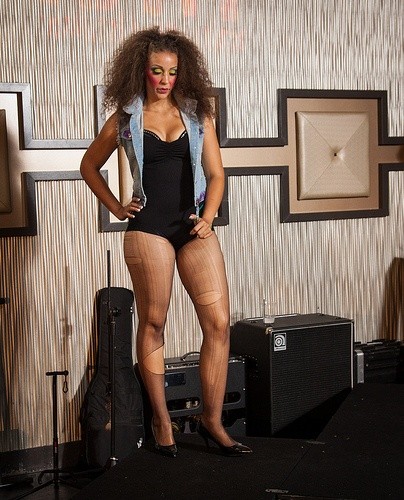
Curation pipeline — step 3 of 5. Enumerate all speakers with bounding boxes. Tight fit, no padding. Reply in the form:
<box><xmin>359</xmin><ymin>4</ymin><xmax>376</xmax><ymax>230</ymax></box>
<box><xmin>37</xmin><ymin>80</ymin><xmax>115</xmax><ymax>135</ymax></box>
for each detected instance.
<box><xmin>235</xmin><ymin>313</ymin><xmax>354</xmax><ymax>442</ymax></box>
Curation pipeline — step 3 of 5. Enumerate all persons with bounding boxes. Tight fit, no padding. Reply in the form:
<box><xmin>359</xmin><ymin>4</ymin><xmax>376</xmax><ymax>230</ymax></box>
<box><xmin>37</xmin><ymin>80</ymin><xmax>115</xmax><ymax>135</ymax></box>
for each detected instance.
<box><xmin>80</xmin><ymin>26</ymin><xmax>253</xmax><ymax>458</ymax></box>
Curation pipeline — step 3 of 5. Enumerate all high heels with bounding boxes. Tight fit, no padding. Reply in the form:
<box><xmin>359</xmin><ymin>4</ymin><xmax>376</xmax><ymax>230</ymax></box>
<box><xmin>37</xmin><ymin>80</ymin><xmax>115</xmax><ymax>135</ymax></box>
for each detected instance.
<box><xmin>196</xmin><ymin>418</ymin><xmax>254</xmax><ymax>457</ymax></box>
<box><xmin>151</xmin><ymin>417</ymin><xmax>178</xmax><ymax>458</ymax></box>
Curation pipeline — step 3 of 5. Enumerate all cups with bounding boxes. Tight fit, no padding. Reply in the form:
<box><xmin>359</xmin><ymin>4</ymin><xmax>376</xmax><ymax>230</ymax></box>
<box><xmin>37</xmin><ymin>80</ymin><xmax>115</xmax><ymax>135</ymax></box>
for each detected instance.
<box><xmin>262</xmin><ymin>302</ymin><xmax>277</xmax><ymax>323</ymax></box>
